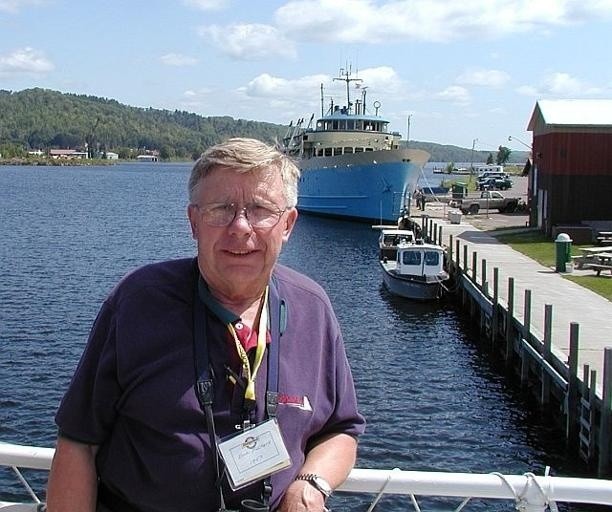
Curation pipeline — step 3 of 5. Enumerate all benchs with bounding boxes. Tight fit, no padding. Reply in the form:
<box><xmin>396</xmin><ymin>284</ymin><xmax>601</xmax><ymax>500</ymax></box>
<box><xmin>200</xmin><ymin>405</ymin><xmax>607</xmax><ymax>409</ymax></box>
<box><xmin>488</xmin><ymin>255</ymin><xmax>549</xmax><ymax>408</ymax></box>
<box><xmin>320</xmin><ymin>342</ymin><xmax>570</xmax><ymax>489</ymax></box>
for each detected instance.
<box><xmin>570</xmin><ymin>253</ymin><xmax>612</xmax><ymax>277</ymax></box>
<box><xmin>596</xmin><ymin>236</ymin><xmax>612</xmax><ymax>244</ymax></box>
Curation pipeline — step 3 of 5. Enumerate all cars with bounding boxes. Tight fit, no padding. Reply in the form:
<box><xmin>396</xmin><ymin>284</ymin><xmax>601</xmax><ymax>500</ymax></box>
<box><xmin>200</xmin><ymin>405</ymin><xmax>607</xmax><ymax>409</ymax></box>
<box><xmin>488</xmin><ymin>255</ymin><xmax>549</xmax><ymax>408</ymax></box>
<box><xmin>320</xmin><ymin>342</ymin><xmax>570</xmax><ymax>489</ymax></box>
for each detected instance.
<box><xmin>471</xmin><ymin>164</ymin><xmax>513</xmax><ymax>192</ymax></box>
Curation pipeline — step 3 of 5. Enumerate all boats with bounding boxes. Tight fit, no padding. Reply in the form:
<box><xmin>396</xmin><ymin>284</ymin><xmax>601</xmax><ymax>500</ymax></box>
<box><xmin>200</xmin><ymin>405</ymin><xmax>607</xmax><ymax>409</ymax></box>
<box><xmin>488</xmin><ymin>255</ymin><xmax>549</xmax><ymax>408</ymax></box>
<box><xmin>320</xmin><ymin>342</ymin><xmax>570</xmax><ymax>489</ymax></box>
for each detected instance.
<box><xmin>276</xmin><ymin>64</ymin><xmax>432</xmax><ymax>226</ymax></box>
<box><xmin>377</xmin><ymin>228</ymin><xmax>451</xmax><ymax>300</ymax></box>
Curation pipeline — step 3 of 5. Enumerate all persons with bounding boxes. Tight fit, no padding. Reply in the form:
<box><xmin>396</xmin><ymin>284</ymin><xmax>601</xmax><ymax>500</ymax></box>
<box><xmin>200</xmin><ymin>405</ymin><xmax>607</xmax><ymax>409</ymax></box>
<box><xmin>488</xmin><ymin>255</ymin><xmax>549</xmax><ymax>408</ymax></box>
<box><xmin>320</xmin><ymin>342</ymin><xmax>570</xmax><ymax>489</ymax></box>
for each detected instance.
<box><xmin>413</xmin><ymin>188</ymin><xmax>426</xmax><ymax>211</ymax></box>
<box><xmin>44</xmin><ymin>133</ymin><xmax>368</xmax><ymax>511</ymax></box>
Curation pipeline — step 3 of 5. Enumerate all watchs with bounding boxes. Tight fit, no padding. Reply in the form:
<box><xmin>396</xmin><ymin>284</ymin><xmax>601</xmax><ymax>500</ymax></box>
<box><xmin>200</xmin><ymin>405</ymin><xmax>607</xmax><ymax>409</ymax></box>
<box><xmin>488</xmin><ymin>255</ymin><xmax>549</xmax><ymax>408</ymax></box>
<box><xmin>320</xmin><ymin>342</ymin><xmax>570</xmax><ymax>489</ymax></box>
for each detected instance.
<box><xmin>294</xmin><ymin>471</ymin><xmax>335</xmax><ymax>501</ymax></box>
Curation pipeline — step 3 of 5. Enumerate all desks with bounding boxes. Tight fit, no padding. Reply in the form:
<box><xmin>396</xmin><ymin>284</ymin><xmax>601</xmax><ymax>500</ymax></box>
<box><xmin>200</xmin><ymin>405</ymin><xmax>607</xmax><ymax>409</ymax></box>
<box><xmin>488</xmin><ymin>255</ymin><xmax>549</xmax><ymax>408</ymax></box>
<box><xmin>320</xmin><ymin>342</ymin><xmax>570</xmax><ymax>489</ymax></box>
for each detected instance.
<box><xmin>577</xmin><ymin>246</ymin><xmax>611</xmax><ymax>253</ymax></box>
<box><xmin>598</xmin><ymin>231</ymin><xmax>612</xmax><ymax>235</ymax></box>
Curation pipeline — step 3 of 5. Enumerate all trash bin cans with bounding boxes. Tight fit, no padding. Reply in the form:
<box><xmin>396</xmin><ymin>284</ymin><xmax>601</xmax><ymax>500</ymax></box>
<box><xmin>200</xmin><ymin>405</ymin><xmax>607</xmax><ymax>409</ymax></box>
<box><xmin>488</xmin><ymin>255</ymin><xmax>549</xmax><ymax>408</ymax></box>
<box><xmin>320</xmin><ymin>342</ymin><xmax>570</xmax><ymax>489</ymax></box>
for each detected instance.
<box><xmin>452</xmin><ymin>182</ymin><xmax>468</xmax><ymax>202</ymax></box>
<box><xmin>555</xmin><ymin>232</ymin><xmax>574</xmax><ymax>273</ymax></box>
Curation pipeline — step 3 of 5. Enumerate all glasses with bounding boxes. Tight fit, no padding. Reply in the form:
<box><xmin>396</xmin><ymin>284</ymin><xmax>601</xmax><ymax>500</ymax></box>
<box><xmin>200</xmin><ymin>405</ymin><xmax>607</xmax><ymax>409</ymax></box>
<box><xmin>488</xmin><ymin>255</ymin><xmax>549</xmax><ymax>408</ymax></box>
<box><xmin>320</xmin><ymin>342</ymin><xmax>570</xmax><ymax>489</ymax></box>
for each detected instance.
<box><xmin>196</xmin><ymin>203</ymin><xmax>288</xmax><ymax>229</ymax></box>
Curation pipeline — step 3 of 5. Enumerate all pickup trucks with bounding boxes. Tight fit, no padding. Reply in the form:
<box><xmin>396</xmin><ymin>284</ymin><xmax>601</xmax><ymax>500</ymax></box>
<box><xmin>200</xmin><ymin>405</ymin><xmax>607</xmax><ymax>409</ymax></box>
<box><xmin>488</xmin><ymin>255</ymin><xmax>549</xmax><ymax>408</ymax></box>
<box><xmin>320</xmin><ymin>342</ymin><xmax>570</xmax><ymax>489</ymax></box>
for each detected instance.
<box><xmin>451</xmin><ymin>190</ymin><xmax>518</xmax><ymax>215</ymax></box>
